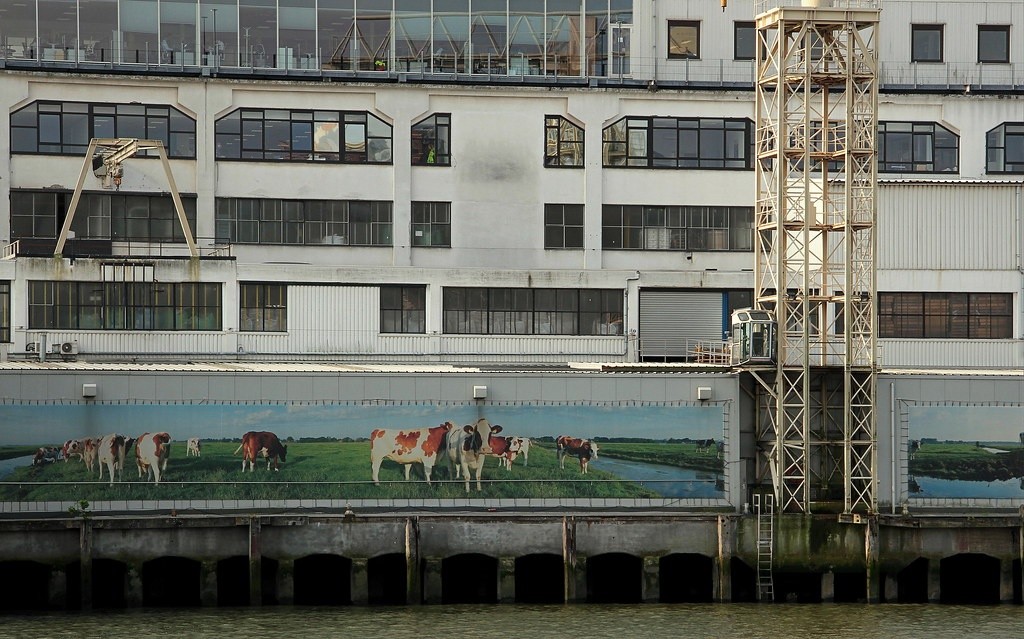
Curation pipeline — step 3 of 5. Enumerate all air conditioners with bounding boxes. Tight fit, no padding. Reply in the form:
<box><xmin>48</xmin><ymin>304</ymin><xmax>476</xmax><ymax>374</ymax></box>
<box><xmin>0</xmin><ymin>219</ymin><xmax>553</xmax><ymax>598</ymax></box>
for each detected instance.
<box><xmin>34</xmin><ymin>341</ymin><xmax>52</xmax><ymax>353</ymax></box>
<box><xmin>60</xmin><ymin>342</ymin><xmax>78</xmax><ymax>355</ymax></box>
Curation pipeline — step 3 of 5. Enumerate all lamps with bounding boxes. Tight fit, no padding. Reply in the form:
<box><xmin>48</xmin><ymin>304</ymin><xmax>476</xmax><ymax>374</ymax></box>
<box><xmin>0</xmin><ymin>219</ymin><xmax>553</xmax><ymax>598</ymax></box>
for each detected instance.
<box><xmin>697</xmin><ymin>386</ymin><xmax>712</xmax><ymax>400</ymax></box>
<box><xmin>82</xmin><ymin>384</ymin><xmax>97</xmax><ymax>397</ymax></box>
<box><xmin>473</xmin><ymin>386</ymin><xmax>487</xmax><ymax>398</ymax></box>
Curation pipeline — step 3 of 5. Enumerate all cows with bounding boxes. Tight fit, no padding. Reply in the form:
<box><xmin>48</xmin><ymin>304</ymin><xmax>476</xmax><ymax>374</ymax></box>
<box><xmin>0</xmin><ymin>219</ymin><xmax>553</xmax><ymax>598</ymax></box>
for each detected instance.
<box><xmin>695</xmin><ymin>438</ymin><xmax>725</xmax><ymax>459</ymax></box>
<box><xmin>370</xmin><ymin>418</ymin><xmax>534</xmax><ymax>493</ymax></box>
<box><xmin>32</xmin><ymin>431</ymin><xmax>173</xmax><ymax>486</ymax></box>
<box><xmin>556</xmin><ymin>435</ymin><xmax>601</xmax><ymax>474</ymax></box>
<box><xmin>234</xmin><ymin>431</ymin><xmax>288</xmax><ymax>474</ymax></box>
<box><xmin>186</xmin><ymin>437</ymin><xmax>202</xmax><ymax>458</ymax></box>
<box><xmin>908</xmin><ymin>473</ymin><xmax>924</xmax><ymax>494</ymax></box>
<box><xmin>908</xmin><ymin>439</ymin><xmax>924</xmax><ymax>461</ymax></box>
